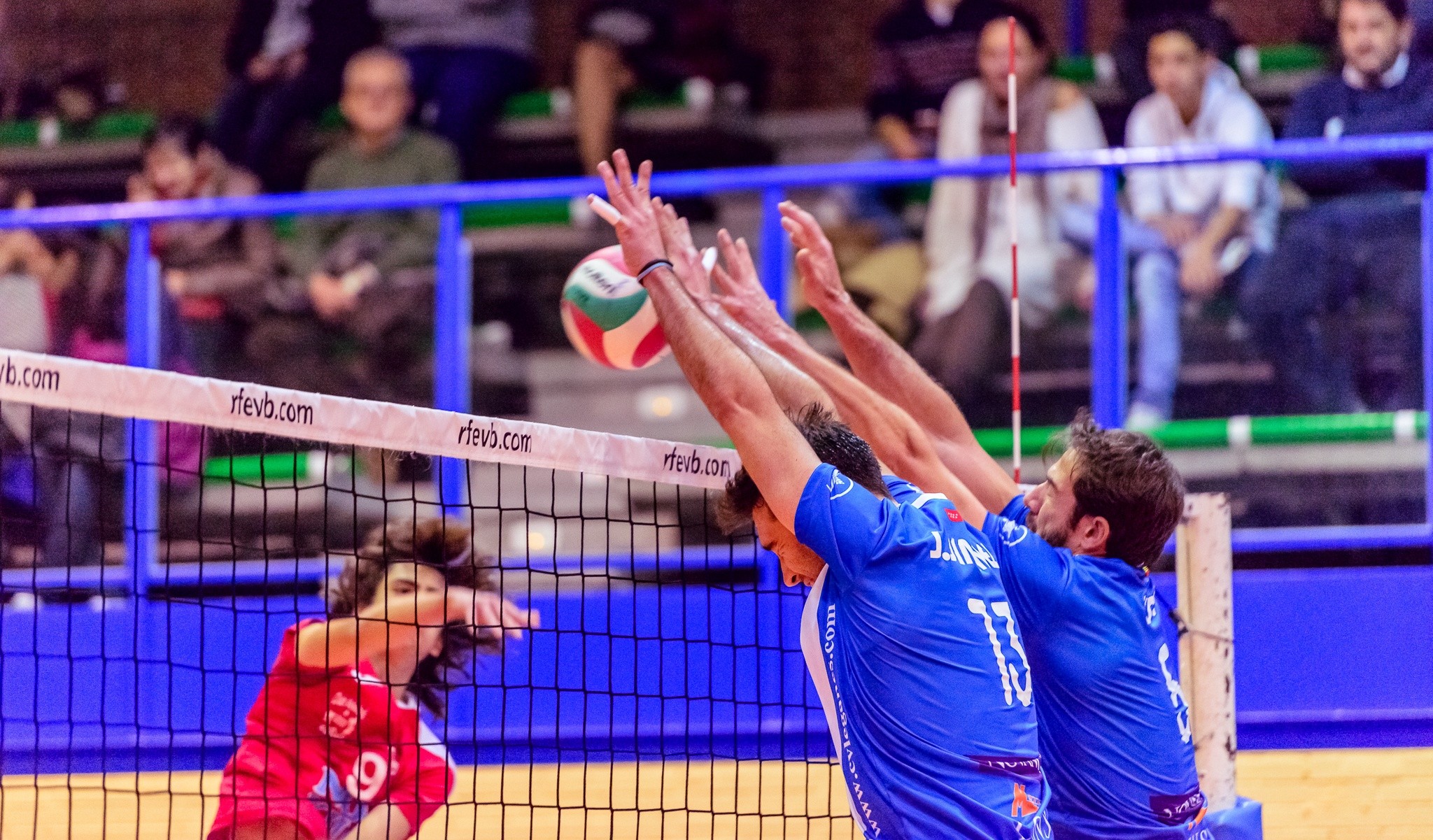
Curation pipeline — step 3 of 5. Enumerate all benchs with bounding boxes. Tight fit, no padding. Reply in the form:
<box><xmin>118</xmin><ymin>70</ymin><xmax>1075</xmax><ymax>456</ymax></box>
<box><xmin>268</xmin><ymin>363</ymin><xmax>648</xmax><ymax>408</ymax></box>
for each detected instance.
<box><xmin>0</xmin><ymin>0</ymin><xmax>1433</xmax><ymax>548</ymax></box>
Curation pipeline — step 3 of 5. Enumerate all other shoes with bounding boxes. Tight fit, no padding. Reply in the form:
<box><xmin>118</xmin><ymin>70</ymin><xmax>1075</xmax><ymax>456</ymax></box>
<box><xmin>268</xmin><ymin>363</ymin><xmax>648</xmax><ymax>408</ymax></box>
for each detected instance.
<box><xmin>360</xmin><ymin>442</ymin><xmax>439</xmax><ymax>483</ymax></box>
<box><xmin>1125</xmin><ymin>398</ymin><xmax>1170</xmax><ymax>432</ymax></box>
<box><xmin>1215</xmin><ymin>235</ymin><xmax>1254</xmax><ymax>278</ymax></box>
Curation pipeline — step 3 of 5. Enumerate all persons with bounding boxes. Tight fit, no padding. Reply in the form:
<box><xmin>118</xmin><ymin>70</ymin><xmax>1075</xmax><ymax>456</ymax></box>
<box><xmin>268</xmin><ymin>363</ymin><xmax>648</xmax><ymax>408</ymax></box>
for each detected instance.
<box><xmin>703</xmin><ymin>200</ymin><xmax>1230</xmax><ymax>840</ymax></box>
<box><xmin>206</xmin><ymin>509</ymin><xmax>539</xmax><ymax>840</ymax></box>
<box><xmin>585</xmin><ymin>146</ymin><xmax>1058</xmax><ymax>840</ymax></box>
<box><xmin>0</xmin><ymin>0</ymin><xmax>560</xmax><ymax>618</ymax></box>
<box><xmin>575</xmin><ymin>0</ymin><xmax>779</xmax><ymax>168</ymax></box>
<box><xmin>817</xmin><ymin>1</ymin><xmax>1430</xmax><ymax>433</ymax></box>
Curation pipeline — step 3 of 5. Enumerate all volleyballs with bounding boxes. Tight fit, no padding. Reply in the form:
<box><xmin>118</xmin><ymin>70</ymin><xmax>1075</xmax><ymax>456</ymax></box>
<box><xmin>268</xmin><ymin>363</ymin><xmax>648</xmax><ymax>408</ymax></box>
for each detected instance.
<box><xmin>560</xmin><ymin>245</ymin><xmax>674</xmax><ymax>369</ymax></box>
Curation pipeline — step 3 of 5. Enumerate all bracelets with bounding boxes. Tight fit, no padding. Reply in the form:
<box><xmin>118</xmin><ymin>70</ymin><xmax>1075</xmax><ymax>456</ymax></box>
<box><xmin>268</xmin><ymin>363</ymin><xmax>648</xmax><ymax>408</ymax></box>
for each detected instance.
<box><xmin>636</xmin><ymin>259</ymin><xmax>676</xmax><ymax>284</ymax></box>
